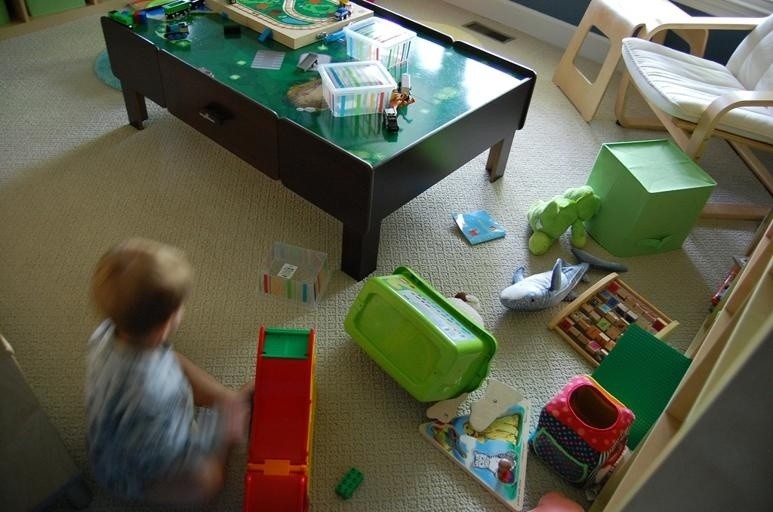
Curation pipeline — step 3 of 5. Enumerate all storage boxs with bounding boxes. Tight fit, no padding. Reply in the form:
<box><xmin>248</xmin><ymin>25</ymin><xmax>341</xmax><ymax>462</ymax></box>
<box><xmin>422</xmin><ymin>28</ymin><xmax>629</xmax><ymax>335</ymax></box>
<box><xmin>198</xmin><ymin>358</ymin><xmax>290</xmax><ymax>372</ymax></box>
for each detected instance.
<box><xmin>257</xmin><ymin>242</ymin><xmax>330</xmax><ymax>309</ymax></box>
<box><xmin>318</xmin><ymin>59</ymin><xmax>397</xmax><ymax>119</ymax></box>
<box><xmin>343</xmin><ymin>17</ymin><xmax>417</xmax><ymax>70</ymax></box>
<box><xmin>581</xmin><ymin>139</ymin><xmax>717</xmax><ymax>257</ymax></box>
<box><xmin>343</xmin><ymin>267</ymin><xmax>497</xmax><ymax>403</ymax></box>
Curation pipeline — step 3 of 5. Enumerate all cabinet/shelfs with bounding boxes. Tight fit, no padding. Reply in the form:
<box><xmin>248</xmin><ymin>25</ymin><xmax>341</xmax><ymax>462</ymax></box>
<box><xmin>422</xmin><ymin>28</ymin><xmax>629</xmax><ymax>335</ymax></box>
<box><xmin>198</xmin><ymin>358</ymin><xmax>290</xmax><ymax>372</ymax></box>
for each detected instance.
<box><xmin>587</xmin><ymin>206</ymin><xmax>773</xmax><ymax>512</ymax></box>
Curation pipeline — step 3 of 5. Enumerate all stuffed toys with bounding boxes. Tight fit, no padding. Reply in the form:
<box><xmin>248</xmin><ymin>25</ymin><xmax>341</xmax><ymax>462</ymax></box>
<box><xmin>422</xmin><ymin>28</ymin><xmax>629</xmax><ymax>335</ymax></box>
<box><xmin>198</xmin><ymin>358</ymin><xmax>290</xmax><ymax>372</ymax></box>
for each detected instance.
<box><xmin>526</xmin><ymin>185</ymin><xmax>601</xmax><ymax>255</ymax></box>
<box><xmin>499</xmin><ymin>247</ymin><xmax>628</xmax><ymax>311</ymax></box>
<box><xmin>444</xmin><ymin>293</ymin><xmax>488</xmax><ymax>328</ymax></box>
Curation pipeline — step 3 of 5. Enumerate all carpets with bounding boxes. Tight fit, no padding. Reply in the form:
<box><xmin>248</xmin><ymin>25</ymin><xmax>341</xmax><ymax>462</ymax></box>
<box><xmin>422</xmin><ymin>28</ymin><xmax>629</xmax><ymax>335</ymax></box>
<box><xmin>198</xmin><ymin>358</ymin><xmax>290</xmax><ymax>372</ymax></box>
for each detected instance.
<box><xmin>95</xmin><ymin>48</ymin><xmax>121</xmax><ymax>91</ymax></box>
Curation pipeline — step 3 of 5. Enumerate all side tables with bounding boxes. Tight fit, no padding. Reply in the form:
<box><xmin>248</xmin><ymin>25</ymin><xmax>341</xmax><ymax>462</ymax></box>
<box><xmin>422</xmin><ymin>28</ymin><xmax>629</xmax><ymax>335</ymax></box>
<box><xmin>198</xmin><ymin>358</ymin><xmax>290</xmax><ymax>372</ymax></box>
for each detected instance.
<box><xmin>552</xmin><ymin>0</ymin><xmax>708</xmax><ymax>123</ymax></box>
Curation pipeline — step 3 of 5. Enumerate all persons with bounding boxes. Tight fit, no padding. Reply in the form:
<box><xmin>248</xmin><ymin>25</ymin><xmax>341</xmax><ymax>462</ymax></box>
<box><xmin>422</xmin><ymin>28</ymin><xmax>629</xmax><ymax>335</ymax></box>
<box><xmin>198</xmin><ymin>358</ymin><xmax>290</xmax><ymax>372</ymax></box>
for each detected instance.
<box><xmin>83</xmin><ymin>235</ymin><xmax>256</xmax><ymax>509</ymax></box>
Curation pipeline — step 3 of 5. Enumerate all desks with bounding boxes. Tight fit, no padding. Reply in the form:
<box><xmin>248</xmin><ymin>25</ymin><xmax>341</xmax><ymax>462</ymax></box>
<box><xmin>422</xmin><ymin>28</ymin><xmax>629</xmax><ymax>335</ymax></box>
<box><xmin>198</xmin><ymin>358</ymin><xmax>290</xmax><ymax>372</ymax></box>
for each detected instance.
<box><xmin>99</xmin><ymin>0</ymin><xmax>538</xmax><ymax>283</ymax></box>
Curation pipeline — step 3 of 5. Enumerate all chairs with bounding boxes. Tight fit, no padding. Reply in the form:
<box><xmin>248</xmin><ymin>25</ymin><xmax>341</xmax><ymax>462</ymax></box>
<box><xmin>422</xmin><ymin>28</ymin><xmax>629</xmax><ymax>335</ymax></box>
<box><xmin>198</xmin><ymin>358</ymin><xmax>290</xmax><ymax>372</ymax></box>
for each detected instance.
<box><xmin>615</xmin><ymin>12</ymin><xmax>773</xmax><ymax>221</ymax></box>
